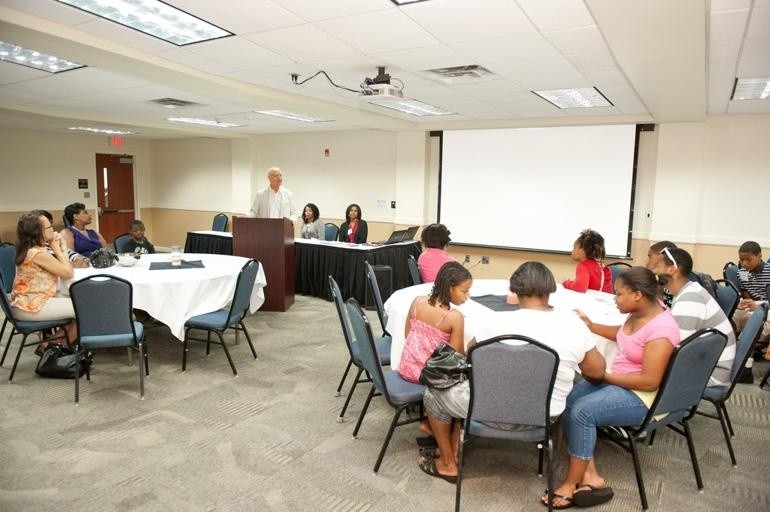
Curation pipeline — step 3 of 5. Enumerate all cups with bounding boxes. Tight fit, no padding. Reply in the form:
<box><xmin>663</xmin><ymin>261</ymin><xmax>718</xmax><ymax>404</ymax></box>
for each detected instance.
<box><xmin>171</xmin><ymin>246</ymin><xmax>181</xmax><ymax>266</ymax></box>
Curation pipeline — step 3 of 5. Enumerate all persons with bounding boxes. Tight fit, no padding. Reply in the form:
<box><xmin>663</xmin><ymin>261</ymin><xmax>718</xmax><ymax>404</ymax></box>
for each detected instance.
<box><xmin>339</xmin><ymin>204</ymin><xmax>368</xmax><ymax>243</ymax></box>
<box><xmin>299</xmin><ymin>203</ymin><xmax>326</xmax><ymax>239</ymax></box>
<box><xmin>731</xmin><ymin>241</ymin><xmax>770</xmax><ymax>384</ymax></box>
<box><xmin>399</xmin><ymin>261</ymin><xmax>473</xmax><ymax>438</ymax></box>
<box><xmin>41</xmin><ymin>209</ymin><xmax>89</xmax><ymax>268</ymax></box>
<box><xmin>250</xmin><ymin>167</ymin><xmax>299</xmax><ymax>226</ymax></box>
<box><xmin>564</xmin><ymin>229</ymin><xmax>614</xmax><ymax>294</ymax></box>
<box><xmin>58</xmin><ymin>202</ymin><xmax>107</xmax><ymax>258</ymax></box>
<box><xmin>421</xmin><ymin>223</ymin><xmax>457</xmax><ymax>284</ymax></box>
<box><xmin>540</xmin><ymin>507</ymin><xmax>541</xmax><ymax>509</ymax></box>
<box><xmin>417</xmin><ymin>261</ymin><xmax>606</xmax><ymax>484</ymax></box>
<box><xmin>123</xmin><ymin>220</ymin><xmax>153</xmax><ymax>253</ymax></box>
<box><xmin>9</xmin><ymin>212</ymin><xmax>79</xmax><ymax>357</ymax></box>
<box><xmin>648</xmin><ymin>239</ymin><xmax>736</xmax><ymax>400</ymax></box>
<box><xmin>544</xmin><ymin>266</ymin><xmax>682</xmax><ymax>508</ymax></box>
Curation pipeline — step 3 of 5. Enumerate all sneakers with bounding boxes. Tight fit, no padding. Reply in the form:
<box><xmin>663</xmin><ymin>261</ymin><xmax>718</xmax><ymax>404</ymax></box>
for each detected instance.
<box><xmin>35</xmin><ymin>344</ymin><xmax>45</xmax><ymax>356</ymax></box>
<box><xmin>736</xmin><ymin>367</ymin><xmax>753</xmax><ymax>384</ymax></box>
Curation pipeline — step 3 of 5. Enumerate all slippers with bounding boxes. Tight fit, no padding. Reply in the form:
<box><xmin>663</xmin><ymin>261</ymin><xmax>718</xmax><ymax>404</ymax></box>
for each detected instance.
<box><xmin>542</xmin><ymin>488</ymin><xmax>573</xmax><ymax>509</ymax></box>
<box><xmin>573</xmin><ymin>484</ymin><xmax>614</xmax><ymax>506</ymax></box>
<box><xmin>416</xmin><ymin>435</ymin><xmax>457</xmax><ymax>483</ymax></box>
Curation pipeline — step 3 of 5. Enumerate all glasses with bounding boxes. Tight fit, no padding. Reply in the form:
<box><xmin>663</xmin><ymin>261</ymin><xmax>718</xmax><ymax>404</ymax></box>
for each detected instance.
<box><xmin>660</xmin><ymin>247</ymin><xmax>678</xmax><ymax>269</ymax></box>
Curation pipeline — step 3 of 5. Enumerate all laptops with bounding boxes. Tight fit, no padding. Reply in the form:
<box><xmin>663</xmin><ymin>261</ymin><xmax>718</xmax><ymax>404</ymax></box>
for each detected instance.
<box><xmin>371</xmin><ymin>226</ymin><xmax>408</xmax><ymax>244</ymax></box>
<box><xmin>402</xmin><ymin>226</ymin><xmax>420</xmax><ymax>241</ymax></box>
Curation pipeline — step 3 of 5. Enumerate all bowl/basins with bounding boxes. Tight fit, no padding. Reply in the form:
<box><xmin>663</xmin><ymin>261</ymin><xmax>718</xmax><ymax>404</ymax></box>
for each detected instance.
<box><xmin>113</xmin><ymin>253</ymin><xmax>141</xmax><ymax>267</ymax></box>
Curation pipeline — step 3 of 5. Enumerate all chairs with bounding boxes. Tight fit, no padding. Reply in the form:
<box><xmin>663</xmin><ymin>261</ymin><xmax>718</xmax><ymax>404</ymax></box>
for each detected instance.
<box><xmin>599</xmin><ymin>263</ymin><xmax>635</xmax><ymax>295</ymax></box>
<box><xmin>587</xmin><ymin>318</ymin><xmax>728</xmax><ymax>511</ymax></box>
<box><xmin>114</xmin><ymin>233</ymin><xmax>147</xmax><ymax>253</ymax></box>
<box><xmin>324</xmin><ymin>223</ymin><xmax>337</xmax><ymax>241</ymax></box>
<box><xmin>1</xmin><ymin>240</ymin><xmax>92</xmax><ymax>386</ymax></box>
<box><xmin>329</xmin><ymin>254</ymin><xmax>438</xmax><ymax>474</ymax></box>
<box><xmin>722</xmin><ymin>257</ymin><xmax>748</xmax><ymax>300</ymax></box>
<box><xmin>180</xmin><ymin>258</ymin><xmax>260</xmax><ymax>378</ymax></box>
<box><xmin>212</xmin><ymin>212</ymin><xmax>228</xmax><ymax>233</ymax></box>
<box><xmin>709</xmin><ymin>279</ymin><xmax>742</xmax><ymax>325</ymax></box>
<box><xmin>452</xmin><ymin>334</ymin><xmax>561</xmax><ymax>511</ymax></box>
<box><xmin>645</xmin><ymin>299</ymin><xmax>769</xmax><ymax>470</ymax></box>
<box><xmin>68</xmin><ymin>273</ymin><xmax>150</xmax><ymax>404</ymax></box>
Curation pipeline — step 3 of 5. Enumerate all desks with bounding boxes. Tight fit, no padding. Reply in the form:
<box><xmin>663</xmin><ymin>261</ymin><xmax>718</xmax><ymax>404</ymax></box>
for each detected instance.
<box><xmin>382</xmin><ymin>278</ymin><xmax>634</xmax><ymax>430</ymax></box>
<box><xmin>55</xmin><ymin>251</ymin><xmax>268</xmax><ymax>346</ymax></box>
<box><xmin>184</xmin><ymin>230</ymin><xmax>422</xmax><ymax>306</ymax></box>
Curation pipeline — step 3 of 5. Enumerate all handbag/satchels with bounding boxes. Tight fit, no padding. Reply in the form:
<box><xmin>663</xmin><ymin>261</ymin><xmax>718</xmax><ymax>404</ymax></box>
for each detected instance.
<box><xmin>90</xmin><ymin>247</ymin><xmax>119</xmax><ymax>268</ymax></box>
<box><xmin>35</xmin><ymin>343</ymin><xmax>95</xmax><ymax>379</ymax></box>
<box><xmin>419</xmin><ymin>341</ymin><xmax>470</xmax><ymax>390</ymax></box>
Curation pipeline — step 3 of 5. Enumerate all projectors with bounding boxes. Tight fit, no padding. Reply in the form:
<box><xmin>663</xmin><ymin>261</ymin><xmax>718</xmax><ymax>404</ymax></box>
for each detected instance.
<box><xmin>359</xmin><ymin>83</ymin><xmax>403</xmax><ymax>101</ymax></box>
